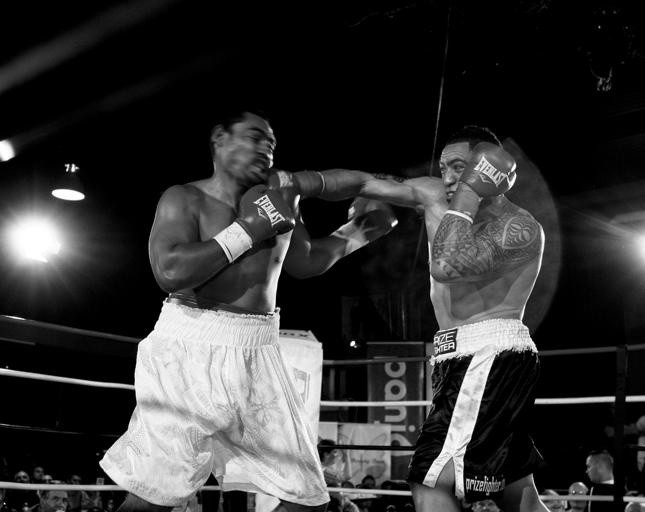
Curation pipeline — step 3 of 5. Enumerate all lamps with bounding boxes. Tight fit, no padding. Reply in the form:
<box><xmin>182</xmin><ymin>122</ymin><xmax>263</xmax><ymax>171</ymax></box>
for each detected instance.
<box><xmin>51</xmin><ymin>164</ymin><xmax>86</xmax><ymax>202</ymax></box>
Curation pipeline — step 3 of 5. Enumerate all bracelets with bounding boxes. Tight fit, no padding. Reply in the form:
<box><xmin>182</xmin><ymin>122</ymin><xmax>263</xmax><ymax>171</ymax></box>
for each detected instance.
<box><xmin>316</xmin><ymin>171</ymin><xmax>326</xmax><ymax>195</ymax></box>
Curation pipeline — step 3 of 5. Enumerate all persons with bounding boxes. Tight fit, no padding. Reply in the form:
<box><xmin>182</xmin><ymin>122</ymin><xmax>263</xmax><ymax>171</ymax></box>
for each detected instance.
<box><xmin>585</xmin><ymin>446</ymin><xmax>631</xmax><ymax>511</ymax></box>
<box><xmin>540</xmin><ymin>489</ymin><xmax>564</xmax><ymax>512</ymax></box>
<box><xmin>264</xmin><ymin>123</ymin><xmax>551</xmax><ymax>512</ymax></box>
<box><xmin>625</xmin><ymin>502</ymin><xmax>645</xmax><ymax>511</ymax></box>
<box><xmin>0</xmin><ymin>467</ymin><xmax>99</xmax><ymax>511</ymax></box>
<box><xmin>568</xmin><ymin>482</ymin><xmax>588</xmax><ymax>511</ymax></box>
<box><xmin>98</xmin><ymin>111</ymin><xmax>400</xmax><ymax>512</ymax></box>
<box><xmin>472</xmin><ymin>499</ymin><xmax>499</xmax><ymax>512</ymax></box>
<box><xmin>318</xmin><ymin>439</ymin><xmax>415</xmax><ymax>512</ymax></box>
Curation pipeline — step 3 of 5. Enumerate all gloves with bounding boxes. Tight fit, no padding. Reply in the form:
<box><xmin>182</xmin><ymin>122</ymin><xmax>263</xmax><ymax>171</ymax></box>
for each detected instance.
<box><xmin>461</xmin><ymin>141</ymin><xmax>518</xmax><ymax>196</ymax></box>
<box><xmin>266</xmin><ymin>168</ymin><xmax>306</xmax><ymax>209</ymax></box>
<box><xmin>346</xmin><ymin>196</ymin><xmax>400</xmax><ymax>243</ymax></box>
<box><xmin>234</xmin><ymin>184</ymin><xmax>296</xmax><ymax>245</ymax></box>
